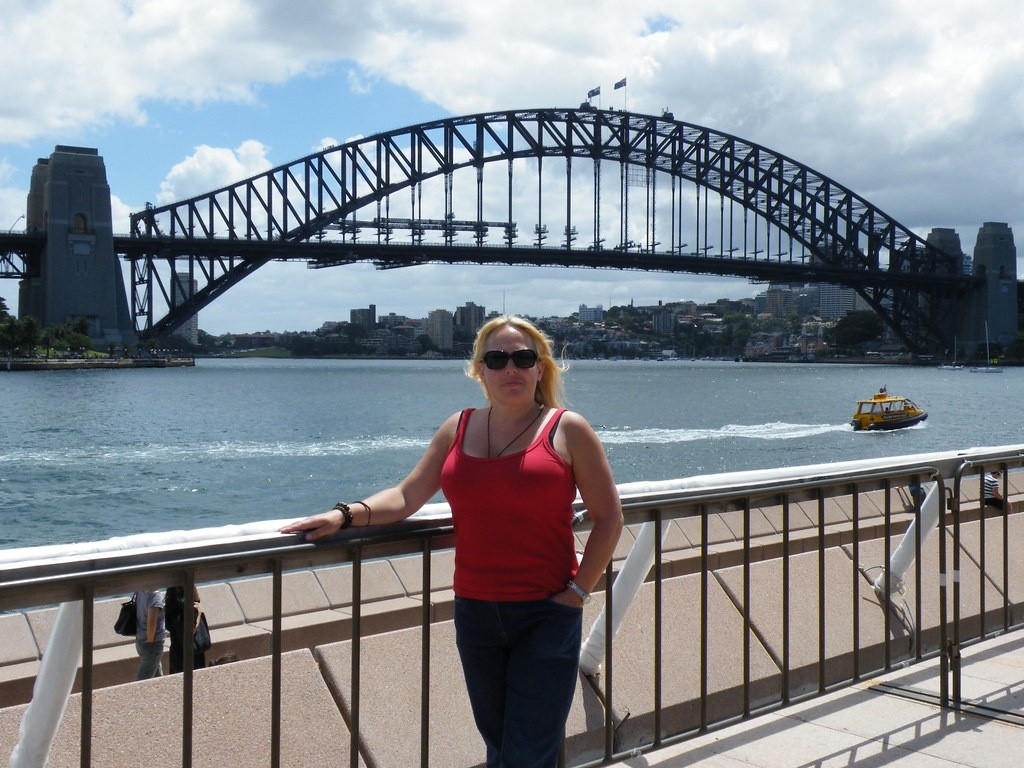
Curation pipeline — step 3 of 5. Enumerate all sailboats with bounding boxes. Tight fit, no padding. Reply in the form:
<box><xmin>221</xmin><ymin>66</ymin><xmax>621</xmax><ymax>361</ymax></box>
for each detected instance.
<box><xmin>969</xmin><ymin>321</ymin><xmax>1002</xmax><ymax>373</ymax></box>
<box><xmin>938</xmin><ymin>336</ymin><xmax>963</xmax><ymax>370</ymax></box>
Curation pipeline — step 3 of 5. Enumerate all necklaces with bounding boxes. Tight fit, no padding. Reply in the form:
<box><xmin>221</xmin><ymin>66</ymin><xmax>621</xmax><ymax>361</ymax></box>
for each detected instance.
<box><xmin>487</xmin><ymin>404</ymin><xmax>545</xmax><ymax>460</ymax></box>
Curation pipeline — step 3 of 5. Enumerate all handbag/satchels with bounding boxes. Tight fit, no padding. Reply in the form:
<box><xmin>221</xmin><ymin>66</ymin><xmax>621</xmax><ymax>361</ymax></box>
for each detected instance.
<box><xmin>113</xmin><ymin>592</ymin><xmax>139</xmax><ymax>636</ymax></box>
<box><xmin>193</xmin><ymin>606</ymin><xmax>211</xmax><ymax>656</ymax></box>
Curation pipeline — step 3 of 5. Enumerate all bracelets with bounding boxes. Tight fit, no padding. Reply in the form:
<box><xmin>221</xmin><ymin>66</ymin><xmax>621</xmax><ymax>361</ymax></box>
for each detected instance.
<box><xmin>333</xmin><ymin>500</ymin><xmax>372</xmax><ymax>527</ymax></box>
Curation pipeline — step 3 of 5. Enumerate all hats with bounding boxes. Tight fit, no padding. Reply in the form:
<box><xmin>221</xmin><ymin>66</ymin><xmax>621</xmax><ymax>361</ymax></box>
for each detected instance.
<box><xmin>997</xmin><ymin>470</ymin><xmax>1002</xmax><ymax>478</ymax></box>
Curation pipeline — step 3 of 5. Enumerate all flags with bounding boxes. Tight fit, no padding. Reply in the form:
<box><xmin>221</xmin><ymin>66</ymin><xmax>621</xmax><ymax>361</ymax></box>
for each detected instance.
<box><xmin>614</xmin><ymin>78</ymin><xmax>626</xmax><ymax>89</ymax></box>
<box><xmin>587</xmin><ymin>86</ymin><xmax>600</xmax><ymax>98</ymax></box>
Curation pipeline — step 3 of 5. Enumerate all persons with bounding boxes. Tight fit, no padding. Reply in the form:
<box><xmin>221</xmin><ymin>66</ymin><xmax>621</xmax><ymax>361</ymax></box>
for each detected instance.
<box><xmin>278</xmin><ymin>314</ymin><xmax>623</xmax><ymax>767</ymax></box>
<box><xmin>982</xmin><ymin>468</ymin><xmax>1014</xmax><ymax>514</ymax></box>
<box><xmin>910</xmin><ymin>483</ymin><xmax>926</xmax><ymax>508</ymax></box>
<box><xmin>114</xmin><ymin>578</ymin><xmax>211</xmax><ymax>681</ymax></box>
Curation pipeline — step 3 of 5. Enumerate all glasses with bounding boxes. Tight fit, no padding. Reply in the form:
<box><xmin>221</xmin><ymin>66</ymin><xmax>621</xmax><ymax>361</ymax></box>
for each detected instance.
<box><xmin>479</xmin><ymin>349</ymin><xmax>542</xmax><ymax>370</ymax></box>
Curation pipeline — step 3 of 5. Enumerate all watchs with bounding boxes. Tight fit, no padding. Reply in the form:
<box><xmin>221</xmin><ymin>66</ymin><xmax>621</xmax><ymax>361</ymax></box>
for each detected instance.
<box><xmin>567</xmin><ymin>581</ymin><xmax>593</xmax><ymax>606</ymax></box>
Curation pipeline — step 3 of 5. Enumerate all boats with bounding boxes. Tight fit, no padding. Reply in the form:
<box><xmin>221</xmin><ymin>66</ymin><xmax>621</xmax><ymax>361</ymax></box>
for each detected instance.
<box><xmin>743</xmin><ymin>347</ymin><xmax>801</xmax><ymax>362</ymax></box>
<box><xmin>849</xmin><ymin>385</ymin><xmax>928</xmax><ymax>431</ymax></box>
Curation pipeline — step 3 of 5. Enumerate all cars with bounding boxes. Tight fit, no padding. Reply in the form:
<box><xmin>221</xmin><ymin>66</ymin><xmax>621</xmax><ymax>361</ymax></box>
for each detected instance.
<box><xmin>19</xmin><ymin>354</ymin><xmax>51</xmax><ymax>358</ymax></box>
